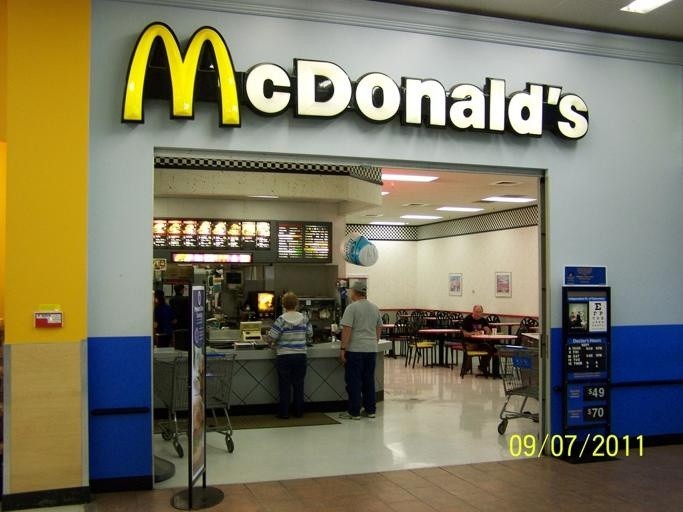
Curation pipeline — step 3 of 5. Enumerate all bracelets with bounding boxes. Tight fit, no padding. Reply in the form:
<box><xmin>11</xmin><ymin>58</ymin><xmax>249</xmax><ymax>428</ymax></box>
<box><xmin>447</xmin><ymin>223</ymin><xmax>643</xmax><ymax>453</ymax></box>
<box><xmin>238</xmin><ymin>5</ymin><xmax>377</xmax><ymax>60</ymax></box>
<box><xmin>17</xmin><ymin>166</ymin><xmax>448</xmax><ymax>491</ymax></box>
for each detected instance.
<box><xmin>340</xmin><ymin>346</ymin><xmax>345</xmax><ymax>350</ymax></box>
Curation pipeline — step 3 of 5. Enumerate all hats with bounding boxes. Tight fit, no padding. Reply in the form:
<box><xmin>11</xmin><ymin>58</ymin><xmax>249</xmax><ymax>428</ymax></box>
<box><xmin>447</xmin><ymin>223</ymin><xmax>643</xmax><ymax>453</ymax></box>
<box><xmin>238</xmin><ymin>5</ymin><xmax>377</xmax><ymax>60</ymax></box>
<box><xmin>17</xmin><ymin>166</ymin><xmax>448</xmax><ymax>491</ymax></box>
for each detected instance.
<box><xmin>351</xmin><ymin>281</ymin><xmax>367</xmax><ymax>292</ymax></box>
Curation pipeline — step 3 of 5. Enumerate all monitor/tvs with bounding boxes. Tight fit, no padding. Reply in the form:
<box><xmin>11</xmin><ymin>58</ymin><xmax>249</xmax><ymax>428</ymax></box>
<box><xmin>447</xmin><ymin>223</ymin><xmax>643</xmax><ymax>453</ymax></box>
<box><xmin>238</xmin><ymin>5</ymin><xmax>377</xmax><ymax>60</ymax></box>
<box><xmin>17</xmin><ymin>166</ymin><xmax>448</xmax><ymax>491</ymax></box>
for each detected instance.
<box><xmin>239</xmin><ymin>321</ymin><xmax>262</xmax><ymax>338</ymax></box>
<box><xmin>225</xmin><ymin>270</ymin><xmax>244</xmax><ymax>286</ymax></box>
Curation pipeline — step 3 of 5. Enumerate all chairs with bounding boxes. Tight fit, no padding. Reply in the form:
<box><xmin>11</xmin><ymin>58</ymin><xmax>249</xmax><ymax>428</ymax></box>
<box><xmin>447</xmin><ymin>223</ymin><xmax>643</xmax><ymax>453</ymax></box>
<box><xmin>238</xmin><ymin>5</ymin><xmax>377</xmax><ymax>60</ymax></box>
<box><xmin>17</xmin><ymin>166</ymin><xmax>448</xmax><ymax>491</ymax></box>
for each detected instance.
<box><xmin>381</xmin><ymin>309</ymin><xmax>538</xmax><ymax>379</ymax></box>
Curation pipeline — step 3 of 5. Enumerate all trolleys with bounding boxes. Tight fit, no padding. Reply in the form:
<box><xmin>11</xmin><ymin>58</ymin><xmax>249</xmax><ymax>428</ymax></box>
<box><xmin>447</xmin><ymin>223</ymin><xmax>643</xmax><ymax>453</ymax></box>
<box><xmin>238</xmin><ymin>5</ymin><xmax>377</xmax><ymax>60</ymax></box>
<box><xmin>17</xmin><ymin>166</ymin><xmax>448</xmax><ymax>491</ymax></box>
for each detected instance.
<box><xmin>494</xmin><ymin>345</ymin><xmax>539</xmax><ymax>440</ymax></box>
<box><xmin>154</xmin><ymin>352</ymin><xmax>237</xmax><ymax>457</ymax></box>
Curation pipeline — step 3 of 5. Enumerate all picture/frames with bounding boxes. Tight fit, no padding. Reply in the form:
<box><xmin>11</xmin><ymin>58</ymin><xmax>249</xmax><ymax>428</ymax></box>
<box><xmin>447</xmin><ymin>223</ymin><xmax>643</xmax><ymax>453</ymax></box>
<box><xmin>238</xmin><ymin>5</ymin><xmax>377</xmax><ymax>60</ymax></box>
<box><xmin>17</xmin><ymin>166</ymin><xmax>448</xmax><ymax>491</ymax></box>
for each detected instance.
<box><xmin>447</xmin><ymin>273</ymin><xmax>462</xmax><ymax>296</ymax></box>
<box><xmin>495</xmin><ymin>272</ymin><xmax>512</xmax><ymax>298</ymax></box>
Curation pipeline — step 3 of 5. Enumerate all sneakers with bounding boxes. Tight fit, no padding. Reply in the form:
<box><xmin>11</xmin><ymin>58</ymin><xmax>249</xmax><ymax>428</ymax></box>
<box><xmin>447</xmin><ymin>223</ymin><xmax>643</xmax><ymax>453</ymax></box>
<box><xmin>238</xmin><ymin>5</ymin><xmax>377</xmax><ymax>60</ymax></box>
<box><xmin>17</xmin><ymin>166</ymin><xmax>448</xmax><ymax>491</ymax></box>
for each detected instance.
<box><xmin>339</xmin><ymin>411</ymin><xmax>361</xmax><ymax>420</ymax></box>
<box><xmin>361</xmin><ymin>411</ymin><xmax>376</xmax><ymax>418</ymax></box>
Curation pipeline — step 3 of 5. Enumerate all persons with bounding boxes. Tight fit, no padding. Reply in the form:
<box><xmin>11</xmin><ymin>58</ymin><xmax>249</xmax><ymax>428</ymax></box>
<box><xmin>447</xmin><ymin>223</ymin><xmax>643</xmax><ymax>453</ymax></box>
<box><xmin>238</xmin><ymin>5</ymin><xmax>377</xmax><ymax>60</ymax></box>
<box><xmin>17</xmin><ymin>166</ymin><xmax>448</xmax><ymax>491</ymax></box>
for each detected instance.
<box><xmin>153</xmin><ymin>289</ymin><xmax>174</xmax><ymax>346</ymax></box>
<box><xmin>460</xmin><ymin>304</ymin><xmax>502</xmax><ymax>378</ymax></box>
<box><xmin>339</xmin><ymin>280</ymin><xmax>381</xmax><ymax>420</ymax></box>
<box><xmin>169</xmin><ymin>284</ymin><xmax>192</xmax><ymax>329</ymax></box>
<box><xmin>264</xmin><ymin>291</ymin><xmax>313</xmax><ymax>419</ymax></box>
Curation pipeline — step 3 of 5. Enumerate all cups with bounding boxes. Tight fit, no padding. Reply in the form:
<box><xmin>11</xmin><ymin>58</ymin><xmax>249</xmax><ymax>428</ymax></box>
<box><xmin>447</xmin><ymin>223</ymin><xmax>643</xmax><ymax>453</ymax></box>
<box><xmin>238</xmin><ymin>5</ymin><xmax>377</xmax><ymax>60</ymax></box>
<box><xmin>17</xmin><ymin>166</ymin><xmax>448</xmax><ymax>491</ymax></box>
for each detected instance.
<box><xmin>331</xmin><ymin>324</ymin><xmax>337</xmax><ymax>336</ymax></box>
<box><xmin>492</xmin><ymin>328</ymin><xmax>497</xmax><ymax>337</ymax></box>
